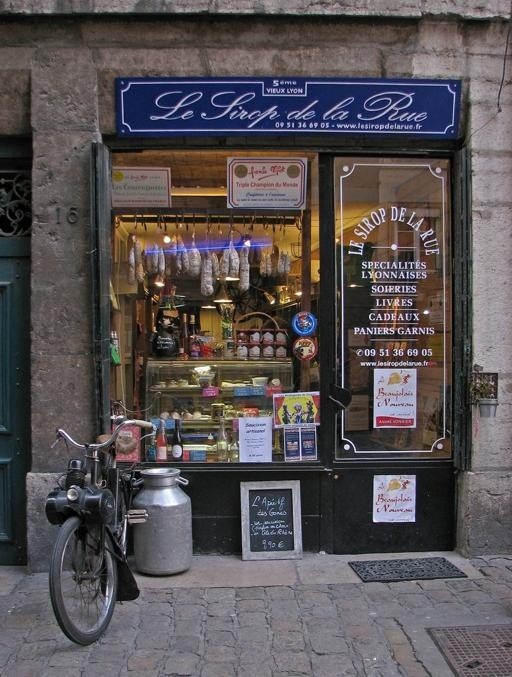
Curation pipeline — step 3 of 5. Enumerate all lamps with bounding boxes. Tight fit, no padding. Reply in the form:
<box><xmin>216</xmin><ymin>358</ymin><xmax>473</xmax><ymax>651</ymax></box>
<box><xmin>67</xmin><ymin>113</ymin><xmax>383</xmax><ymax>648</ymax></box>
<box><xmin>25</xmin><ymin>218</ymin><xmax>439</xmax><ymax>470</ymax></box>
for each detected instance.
<box><xmin>213</xmin><ymin>279</ymin><xmax>232</xmax><ymax>305</ymax></box>
<box><xmin>201</xmin><ymin>294</ymin><xmax>217</xmax><ymax>309</ymax></box>
<box><xmin>346</xmin><ymin>279</ymin><xmax>364</xmax><ymax>289</ymax></box>
<box><xmin>162</xmin><ymin>229</ymin><xmax>170</xmax><ymax>244</ymax></box>
<box><xmin>243</xmin><ymin>235</ymin><xmax>252</xmax><ymax>246</ymax></box>
<box><xmin>294</xmin><ymin>278</ymin><xmax>303</xmax><ymax>296</ymax></box>
<box><xmin>264</xmin><ymin>290</ymin><xmax>276</xmax><ymax>304</ymax></box>
<box><xmin>155</xmin><ymin>273</ymin><xmax>167</xmax><ymax>287</ymax></box>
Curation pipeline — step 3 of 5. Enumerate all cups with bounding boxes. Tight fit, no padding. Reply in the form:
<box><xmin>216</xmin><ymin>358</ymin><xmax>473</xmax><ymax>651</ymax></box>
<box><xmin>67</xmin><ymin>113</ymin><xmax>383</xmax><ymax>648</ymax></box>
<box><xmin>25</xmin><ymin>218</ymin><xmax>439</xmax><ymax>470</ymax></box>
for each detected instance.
<box><xmin>210</xmin><ymin>401</ymin><xmax>226</xmax><ymax>420</ymax></box>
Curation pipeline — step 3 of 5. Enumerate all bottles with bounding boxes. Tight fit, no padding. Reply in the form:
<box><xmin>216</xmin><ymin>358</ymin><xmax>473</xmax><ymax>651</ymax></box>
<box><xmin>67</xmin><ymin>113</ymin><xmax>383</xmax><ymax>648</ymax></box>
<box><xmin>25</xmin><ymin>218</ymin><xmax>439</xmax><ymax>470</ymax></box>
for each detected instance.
<box><xmin>207</xmin><ymin>417</ymin><xmax>239</xmax><ymax>463</ymax></box>
<box><xmin>146</xmin><ymin>419</ymin><xmax>184</xmax><ymax>461</ymax></box>
<box><xmin>177</xmin><ymin>313</ymin><xmax>200</xmax><ymax>356</ymax></box>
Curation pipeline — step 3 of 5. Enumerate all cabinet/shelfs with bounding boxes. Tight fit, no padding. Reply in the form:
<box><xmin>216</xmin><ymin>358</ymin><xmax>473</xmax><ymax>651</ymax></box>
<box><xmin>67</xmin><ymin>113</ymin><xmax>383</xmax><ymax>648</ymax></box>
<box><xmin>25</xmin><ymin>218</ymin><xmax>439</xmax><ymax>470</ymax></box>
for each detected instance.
<box><xmin>146</xmin><ymin>359</ymin><xmax>294</xmax><ymax>451</ymax></box>
<box><xmin>234</xmin><ymin>330</ymin><xmax>291</xmax><ymax>362</ymax></box>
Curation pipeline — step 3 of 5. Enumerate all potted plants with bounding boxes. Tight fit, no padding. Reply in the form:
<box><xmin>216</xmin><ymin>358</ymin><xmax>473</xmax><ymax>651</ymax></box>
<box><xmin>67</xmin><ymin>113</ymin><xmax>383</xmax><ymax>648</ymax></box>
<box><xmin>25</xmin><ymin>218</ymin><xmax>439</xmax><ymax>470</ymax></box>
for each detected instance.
<box><xmin>471</xmin><ymin>375</ymin><xmax>498</xmax><ymax>418</ymax></box>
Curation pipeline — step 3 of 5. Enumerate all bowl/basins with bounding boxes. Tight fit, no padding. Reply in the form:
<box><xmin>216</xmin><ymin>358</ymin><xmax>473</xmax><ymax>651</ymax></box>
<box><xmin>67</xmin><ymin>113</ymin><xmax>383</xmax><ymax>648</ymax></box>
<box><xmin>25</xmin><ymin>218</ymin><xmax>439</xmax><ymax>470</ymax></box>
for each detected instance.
<box><xmin>251</xmin><ymin>376</ymin><xmax>269</xmax><ymax>387</ymax></box>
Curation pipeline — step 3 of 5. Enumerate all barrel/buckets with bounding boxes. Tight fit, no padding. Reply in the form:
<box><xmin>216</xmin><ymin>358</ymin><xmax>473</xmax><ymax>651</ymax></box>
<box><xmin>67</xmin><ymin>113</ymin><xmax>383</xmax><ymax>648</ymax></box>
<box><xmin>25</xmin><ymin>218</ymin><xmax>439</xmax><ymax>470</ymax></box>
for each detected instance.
<box><xmin>131</xmin><ymin>468</ymin><xmax>193</xmax><ymax>577</ymax></box>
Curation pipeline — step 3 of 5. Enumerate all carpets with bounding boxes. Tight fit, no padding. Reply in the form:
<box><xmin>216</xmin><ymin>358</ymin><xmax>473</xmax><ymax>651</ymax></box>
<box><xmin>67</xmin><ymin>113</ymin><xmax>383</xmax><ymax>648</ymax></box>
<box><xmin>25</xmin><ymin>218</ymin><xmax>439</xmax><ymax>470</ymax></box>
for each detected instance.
<box><xmin>345</xmin><ymin>555</ymin><xmax>470</xmax><ymax>583</ymax></box>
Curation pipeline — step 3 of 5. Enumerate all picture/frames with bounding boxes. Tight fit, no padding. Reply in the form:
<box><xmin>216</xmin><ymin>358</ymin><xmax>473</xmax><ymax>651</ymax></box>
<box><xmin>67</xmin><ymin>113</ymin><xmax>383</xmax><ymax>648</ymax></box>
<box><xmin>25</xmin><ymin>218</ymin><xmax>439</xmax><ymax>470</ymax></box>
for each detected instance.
<box><xmin>240</xmin><ymin>478</ymin><xmax>304</xmax><ymax>563</ymax></box>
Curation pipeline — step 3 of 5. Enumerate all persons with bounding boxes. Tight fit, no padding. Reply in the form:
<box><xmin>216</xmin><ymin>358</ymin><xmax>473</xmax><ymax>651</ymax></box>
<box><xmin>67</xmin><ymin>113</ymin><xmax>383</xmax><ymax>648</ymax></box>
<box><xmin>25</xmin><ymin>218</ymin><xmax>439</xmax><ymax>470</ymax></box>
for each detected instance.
<box><xmin>289</xmin><ymin>403</ymin><xmax>306</xmax><ymax>424</ymax></box>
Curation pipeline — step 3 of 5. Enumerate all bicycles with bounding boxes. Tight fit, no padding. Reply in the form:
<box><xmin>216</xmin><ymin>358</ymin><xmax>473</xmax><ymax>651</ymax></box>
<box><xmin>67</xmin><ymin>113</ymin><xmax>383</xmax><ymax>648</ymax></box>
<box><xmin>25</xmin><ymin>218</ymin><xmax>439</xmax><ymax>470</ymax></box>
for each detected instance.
<box><xmin>45</xmin><ymin>415</ymin><xmax>158</xmax><ymax>648</ymax></box>
